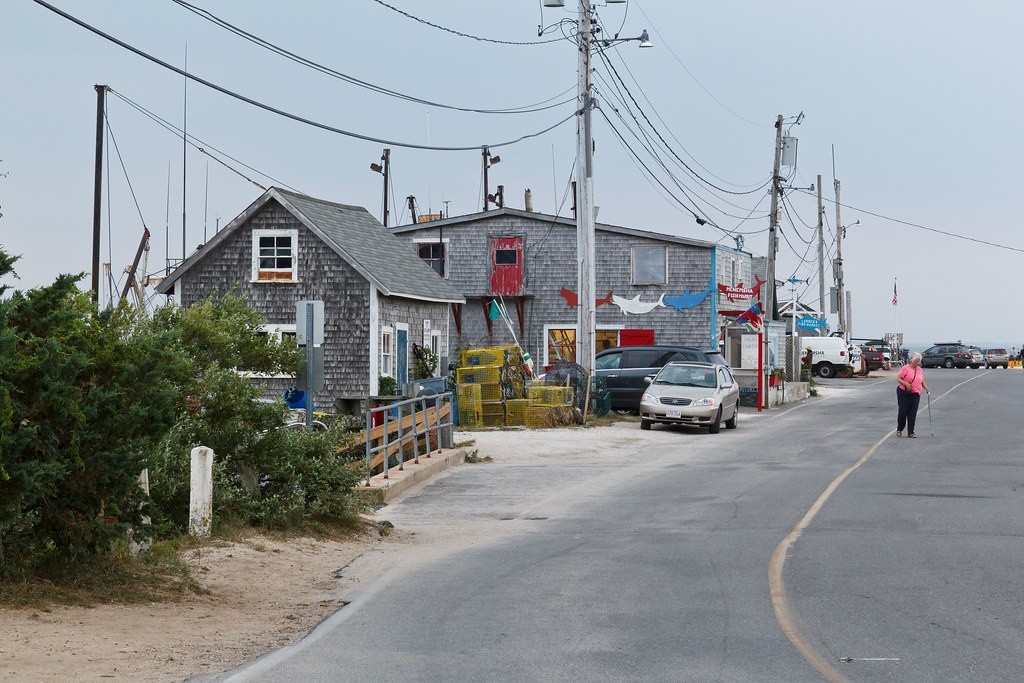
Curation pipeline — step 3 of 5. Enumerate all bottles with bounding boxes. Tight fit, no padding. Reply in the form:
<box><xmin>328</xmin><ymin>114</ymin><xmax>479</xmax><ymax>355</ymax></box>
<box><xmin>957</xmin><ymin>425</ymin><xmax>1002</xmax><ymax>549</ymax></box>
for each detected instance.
<box><xmin>523</xmin><ymin>352</ymin><xmax>534</xmax><ymax>368</ymax></box>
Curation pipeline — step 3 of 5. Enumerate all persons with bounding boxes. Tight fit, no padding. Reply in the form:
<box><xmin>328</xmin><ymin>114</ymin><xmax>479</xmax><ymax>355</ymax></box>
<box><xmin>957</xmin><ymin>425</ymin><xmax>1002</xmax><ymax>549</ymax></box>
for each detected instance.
<box><xmin>1009</xmin><ymin>344</ymin><xmax>1024</xmax><ymax>368</ymax></box>
<box><xmin>896</xmin><ymin>352</ymin><xmax>932</xmax><ymax>438</ymax></box>
<box><xmin>802</xmin><ymin>347</ymin><xmax>812</xmax><ymax>380</ymax></box>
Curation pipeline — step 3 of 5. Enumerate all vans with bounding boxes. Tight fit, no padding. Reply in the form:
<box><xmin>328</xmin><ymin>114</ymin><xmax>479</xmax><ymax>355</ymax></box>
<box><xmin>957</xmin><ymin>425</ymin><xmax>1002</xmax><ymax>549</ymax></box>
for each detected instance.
<box><xmin>798</xmin><ymin>337</ymin><xmax>850</xmax><ymax>378</ymax></box>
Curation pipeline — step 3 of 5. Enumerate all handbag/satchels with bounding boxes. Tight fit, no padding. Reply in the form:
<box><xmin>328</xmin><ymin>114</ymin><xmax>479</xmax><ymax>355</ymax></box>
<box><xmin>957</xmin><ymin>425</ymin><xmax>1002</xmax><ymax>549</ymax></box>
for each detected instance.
<box><xmin>905</xmin><ymin>385</ymin><xmax>912</xmax><ymax>396</ymax></box>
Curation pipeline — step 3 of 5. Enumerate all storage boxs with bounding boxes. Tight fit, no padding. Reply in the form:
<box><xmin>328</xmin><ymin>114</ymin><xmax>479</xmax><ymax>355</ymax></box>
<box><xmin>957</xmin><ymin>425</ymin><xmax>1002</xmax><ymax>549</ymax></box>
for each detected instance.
<box><xmin>457</xmin><ymin>346</ymin><xmax>574</xmax><ymax>428</ymax></box>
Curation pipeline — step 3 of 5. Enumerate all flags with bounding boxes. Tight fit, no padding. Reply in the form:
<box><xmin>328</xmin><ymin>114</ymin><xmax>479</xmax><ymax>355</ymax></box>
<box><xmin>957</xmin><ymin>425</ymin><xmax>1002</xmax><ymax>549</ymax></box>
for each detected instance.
<box><xmin>892</xmin><ymin>281</ymin><xmax>898</xmax><ymax>306</ymax></box>
<box><xmin>489</xmin><ymin>300</ymin><xmax>502</xmax><ymax>321</ymax></box>
<box><xmin>735</xmin><ymin>301</ymin><xmax>763</xmax><ymax>334</ymax></box>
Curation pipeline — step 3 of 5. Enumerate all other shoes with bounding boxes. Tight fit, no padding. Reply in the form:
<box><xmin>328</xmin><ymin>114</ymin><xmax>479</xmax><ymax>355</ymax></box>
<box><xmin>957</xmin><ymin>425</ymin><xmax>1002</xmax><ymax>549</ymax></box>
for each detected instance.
<box><xmin>908</xmin><ymin>433</ymin><xmax>916</xmax><ymax>438</ymax></box>
<box><xmin>897</xmin><ymin>430</ymin><xmax>902</xmax><ymax>436</ymax></box>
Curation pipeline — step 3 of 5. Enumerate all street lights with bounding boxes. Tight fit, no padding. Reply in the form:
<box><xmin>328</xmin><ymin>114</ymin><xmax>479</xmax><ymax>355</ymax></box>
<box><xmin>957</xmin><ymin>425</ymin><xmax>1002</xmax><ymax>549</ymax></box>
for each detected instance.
<box><xmin>759</xmin><ymin>111</ymin><xmax>806</xmax><ymax>403</ymax></box>
<box><xmin>480</xmin><ymin>143</ymin><xmax>502</xmax><ymax>213</ymax></box>
<box><xmin>834</xmin><ymin>219</ymin><xmax>861</xmax><ymax>338</ymax></box>
<box><xmin>571</xmin><ymin>29</ymin><xmax>654</xmax><ymax>369</ymax></box>
<box><xmin>370</xmin><ymin>145</ymin><xmax>394</xmax><ymax>232</ymax></box>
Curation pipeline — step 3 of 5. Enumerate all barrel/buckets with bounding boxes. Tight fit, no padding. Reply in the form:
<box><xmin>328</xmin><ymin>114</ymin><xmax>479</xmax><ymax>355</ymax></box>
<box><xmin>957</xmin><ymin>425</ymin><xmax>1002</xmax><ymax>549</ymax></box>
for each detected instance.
<box><xmin>285</xmin><ymin>386</ymin><xmax>315</xmax><ymax>411</ymax></box>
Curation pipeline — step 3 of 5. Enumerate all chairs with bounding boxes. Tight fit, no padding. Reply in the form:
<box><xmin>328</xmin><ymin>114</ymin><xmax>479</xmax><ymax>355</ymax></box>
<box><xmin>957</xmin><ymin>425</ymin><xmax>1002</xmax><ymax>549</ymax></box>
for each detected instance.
<box><xmin>706</xmin><ymin>373</ymin><xmax>715</xmax><ymax>383</ymax></box>
<box><xmin>672</xmin><ymin>372</ymin><xmax>691</xmax><ymax>380</ymax></box>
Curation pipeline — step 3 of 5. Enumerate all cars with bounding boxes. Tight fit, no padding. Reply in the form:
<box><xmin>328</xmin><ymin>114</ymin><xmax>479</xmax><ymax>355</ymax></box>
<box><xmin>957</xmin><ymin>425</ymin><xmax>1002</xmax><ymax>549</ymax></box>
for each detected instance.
<box><xmin>638</xmin><ymin>361</ymin><xmax>741</xmax><ymax>436</ymax></box>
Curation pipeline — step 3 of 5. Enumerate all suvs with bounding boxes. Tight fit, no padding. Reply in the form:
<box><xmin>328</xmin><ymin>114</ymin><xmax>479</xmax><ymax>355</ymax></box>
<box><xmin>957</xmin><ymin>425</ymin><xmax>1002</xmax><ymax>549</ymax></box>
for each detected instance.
<box><xmin>539</xmin><ymin>345</ymin><xmax>736</xmax><ymax>414</ymax></box>
<box><xmin>968</xmin><ymin>349</ymin><xmax>982</xmax><ymax>369</ymax></box>
<box><xmin>982</xmin><ymin>348</ymin><xmax>1010</xmax><ymax>370</ymax></box>
<box><xmin>917</xmin><ymin>341</ymin><xmax>974</xmax><ymax>368</ymax></box>
<box><xmin>855</xmin><ymin>345</ymin><xmax>884</xmax><ymax>377</ymax></box>
